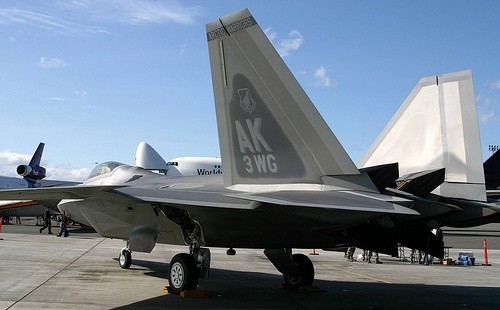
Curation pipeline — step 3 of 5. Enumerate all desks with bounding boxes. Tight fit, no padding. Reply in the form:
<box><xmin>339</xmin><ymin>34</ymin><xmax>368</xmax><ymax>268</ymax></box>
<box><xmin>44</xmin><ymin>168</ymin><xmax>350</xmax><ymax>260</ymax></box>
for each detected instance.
<box><xmin>444</xmin><ymin>247</ymin><xmax>452</xmax><ymax>260</ymax></box>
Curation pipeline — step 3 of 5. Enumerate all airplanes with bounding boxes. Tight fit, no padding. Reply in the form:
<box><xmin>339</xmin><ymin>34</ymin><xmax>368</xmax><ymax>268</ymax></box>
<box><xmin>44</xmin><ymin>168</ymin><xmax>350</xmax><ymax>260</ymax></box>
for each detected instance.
<box><xmin>0</xmin><ymin>142</ymin><xmax>237</xmax><ymax>256</ymax></box>
<box><xmin>0</xmin><ymin>7</ymin><xmax>500</xmax><ymax>296</ymax></box>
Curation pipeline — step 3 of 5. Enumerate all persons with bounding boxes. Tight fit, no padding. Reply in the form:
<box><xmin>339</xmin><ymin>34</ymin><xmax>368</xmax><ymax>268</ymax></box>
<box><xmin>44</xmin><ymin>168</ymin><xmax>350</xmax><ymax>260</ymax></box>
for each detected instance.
<box><xmin>39</xmin><ymin>205</ymin><xmax>70</xmax><ymax>239</ymax></box>
<box><xmin>345</xmin><ymin>243</ymin><xmax>430</xmax><ymax>266</ymax></box>
<box><xmin>434</xmin><ymin>226</ymin><xmax>443</xmax><ymax>241</ymax></box>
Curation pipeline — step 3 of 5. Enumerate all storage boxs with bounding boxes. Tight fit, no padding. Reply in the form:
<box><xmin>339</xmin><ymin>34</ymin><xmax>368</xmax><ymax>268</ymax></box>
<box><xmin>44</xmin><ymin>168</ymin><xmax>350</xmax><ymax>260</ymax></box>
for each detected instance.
<box><xmin>443</xmin><ymin>259</ymin><xmax>452</xmax><ymax>265</ymax></box>
<box><xmin>459</xmin><ymin>251</ymin><xmax>473</xmax><ymax>259</ymax></box>
